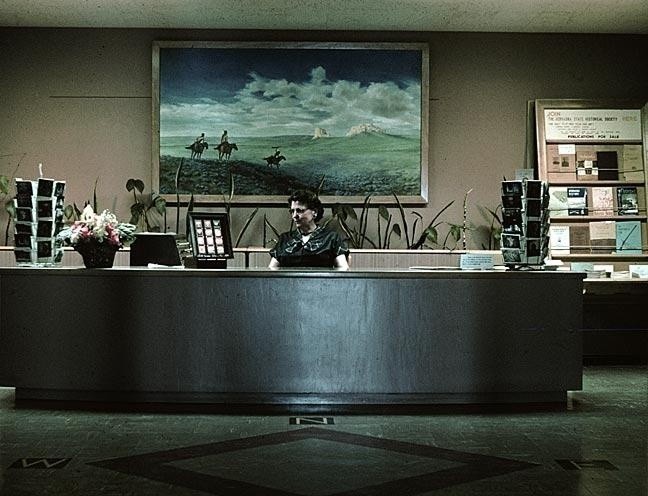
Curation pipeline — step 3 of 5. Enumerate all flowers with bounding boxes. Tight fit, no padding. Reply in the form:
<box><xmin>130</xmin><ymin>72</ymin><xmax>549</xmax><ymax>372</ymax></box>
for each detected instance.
<box><xmin>55</xmin><ymin>204</ymin><xmax>137</xmax><ymax>251</ymax></box>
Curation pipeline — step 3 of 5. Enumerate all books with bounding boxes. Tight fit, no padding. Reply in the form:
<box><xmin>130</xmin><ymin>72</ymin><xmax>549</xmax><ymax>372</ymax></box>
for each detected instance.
<box><xmin>498</xmin><ymin>166</ymin><xmax>551</xmax><ymax>272</ymax></box>
<box><xmin>13</xmin><ymin>177</ymin><xmax>66</xmax><ymax>268</ymax></box>
<box><xmin>546</xmin><ymin>140</ymin><xmax>648</xmax><ymax>280</ymax></box>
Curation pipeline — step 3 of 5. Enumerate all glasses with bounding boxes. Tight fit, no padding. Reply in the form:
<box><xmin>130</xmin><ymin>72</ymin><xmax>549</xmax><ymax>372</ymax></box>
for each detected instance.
<box><xmin>289</xmin><ymin>207</ymin><xmax>309</xmax><ymax>214</ymax></box>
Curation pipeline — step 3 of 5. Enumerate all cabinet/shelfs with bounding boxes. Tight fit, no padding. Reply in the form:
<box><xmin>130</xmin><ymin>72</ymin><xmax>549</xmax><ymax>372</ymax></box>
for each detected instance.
<box><xmin>527</xmin><ymin>99</ymin><xmax>648</xmax><ymax>366</ymax></box>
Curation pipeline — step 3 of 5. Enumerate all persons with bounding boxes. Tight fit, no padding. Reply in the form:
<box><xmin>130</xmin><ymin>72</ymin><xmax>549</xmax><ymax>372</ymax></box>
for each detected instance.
<box><xmin>267</xmin><ymin>188</ymin><xmax>350</xmax><ymax>269</ymax></box>
<box><xmin>274</xmin><ymin>147</ymin><xmax>280</xmax><ymax>160</ymax></box>
<box><xmin>218</xmin><ymin>129</ymin><xmax>229</xmax><ymax>160</ymax></box>
<box><xmin>193</xmin><ymin>132</ymin><xmax>205</xmax><ymax>160</ymax></box>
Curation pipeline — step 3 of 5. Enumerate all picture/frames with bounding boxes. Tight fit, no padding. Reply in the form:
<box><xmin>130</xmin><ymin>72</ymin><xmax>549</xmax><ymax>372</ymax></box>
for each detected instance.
<box><xmin>152</xmin><ymin>41</ymin><xmax>430</xmax><ymax>205</ymax></box>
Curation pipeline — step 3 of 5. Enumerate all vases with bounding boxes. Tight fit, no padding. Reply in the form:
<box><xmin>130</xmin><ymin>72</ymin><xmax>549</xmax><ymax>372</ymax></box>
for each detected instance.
<box><xmin>83</xmin><ymin>248</ymin><xmax>114</xmax><ymax>268</ymax></box>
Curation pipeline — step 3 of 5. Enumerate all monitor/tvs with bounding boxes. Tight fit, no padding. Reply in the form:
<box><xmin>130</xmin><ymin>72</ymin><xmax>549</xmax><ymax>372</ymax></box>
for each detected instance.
<box><xmin>188</xmin><ymin>209</ymin><xmax>234</xmax><ymax>260</ymax></box>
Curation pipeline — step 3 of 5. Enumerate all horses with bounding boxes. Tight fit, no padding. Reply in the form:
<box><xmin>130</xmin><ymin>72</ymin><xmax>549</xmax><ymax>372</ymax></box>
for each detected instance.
<box><xmin>184</xmin><ymin>141</ymin><xmax>209</xmax><ymax>159</ymax></box>
<box><xmin>213</xmin><ymin>142</ymin><xmax>238</xmax><ymax>160</ymax></box>
<box><xmin>262</xmin><ymin>155</ymin><xmax>287</xmax><ymax>169</ymax></box>
